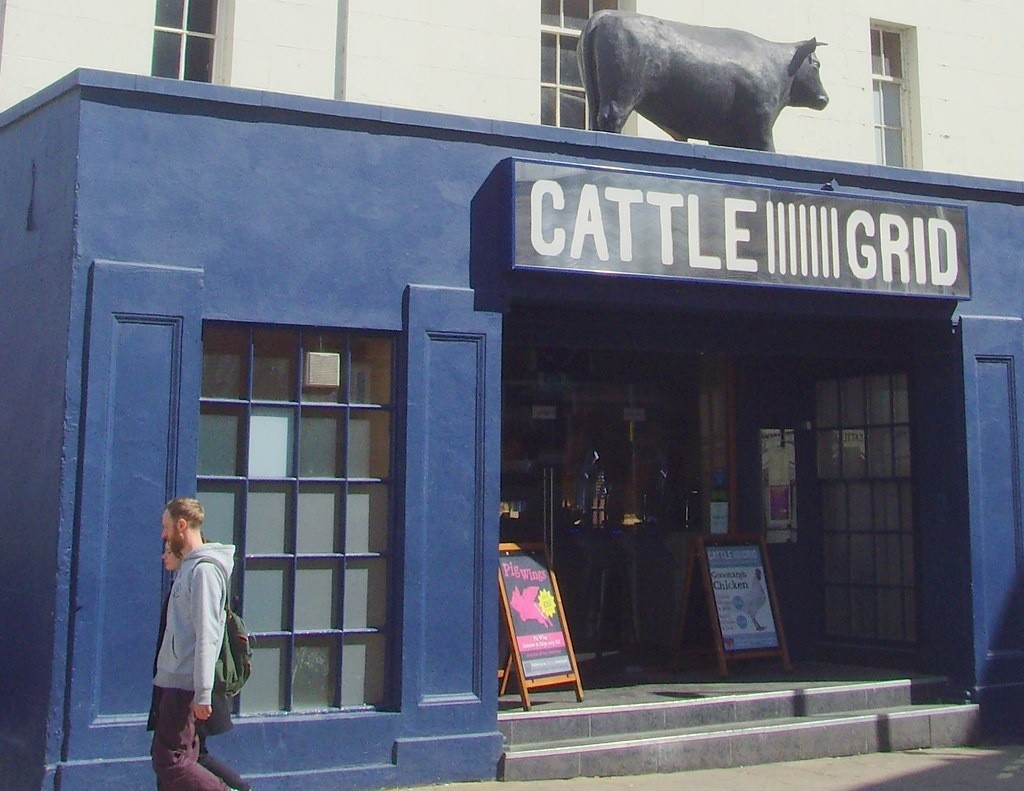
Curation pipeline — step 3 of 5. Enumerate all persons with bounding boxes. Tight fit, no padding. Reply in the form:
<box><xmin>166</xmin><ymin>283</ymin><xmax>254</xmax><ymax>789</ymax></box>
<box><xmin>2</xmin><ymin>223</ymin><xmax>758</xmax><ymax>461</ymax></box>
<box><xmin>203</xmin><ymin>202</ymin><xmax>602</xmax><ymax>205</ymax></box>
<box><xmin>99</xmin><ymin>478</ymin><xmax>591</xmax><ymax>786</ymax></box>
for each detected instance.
<box><xmin>146</xmin><ymin>530</ymin><xmax>253</xmax><ymax>790</ymax></box>
<box><xmin>151</xmin><ymin>497</ymin><xmax>236</xmax><ymax>790</ymax></box>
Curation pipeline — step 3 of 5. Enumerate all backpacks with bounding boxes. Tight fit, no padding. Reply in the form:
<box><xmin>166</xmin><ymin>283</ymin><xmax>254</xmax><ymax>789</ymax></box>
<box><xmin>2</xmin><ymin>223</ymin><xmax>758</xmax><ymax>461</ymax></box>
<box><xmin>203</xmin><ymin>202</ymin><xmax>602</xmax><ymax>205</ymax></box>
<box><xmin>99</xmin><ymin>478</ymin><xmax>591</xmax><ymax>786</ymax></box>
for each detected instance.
<box><xmin>189</xmin><ymin>558</ymin><xmax>255</xmax><ymax>698</ymax></box>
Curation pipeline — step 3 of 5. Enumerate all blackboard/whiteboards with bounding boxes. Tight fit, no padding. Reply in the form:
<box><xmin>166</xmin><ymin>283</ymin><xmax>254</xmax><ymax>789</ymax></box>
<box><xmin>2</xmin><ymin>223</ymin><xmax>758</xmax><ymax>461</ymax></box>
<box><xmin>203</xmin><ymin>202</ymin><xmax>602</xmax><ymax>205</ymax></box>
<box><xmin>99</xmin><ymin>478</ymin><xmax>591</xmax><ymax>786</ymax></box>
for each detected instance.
<box><xmin>673</xmin><ymin>531</ymin><xmax>792</xmax><ymax>680</ymax></box>
<box><xmin>499</xmin><ymin>540</ymin><xmax>583</xmax><ymax>710</ymax></box>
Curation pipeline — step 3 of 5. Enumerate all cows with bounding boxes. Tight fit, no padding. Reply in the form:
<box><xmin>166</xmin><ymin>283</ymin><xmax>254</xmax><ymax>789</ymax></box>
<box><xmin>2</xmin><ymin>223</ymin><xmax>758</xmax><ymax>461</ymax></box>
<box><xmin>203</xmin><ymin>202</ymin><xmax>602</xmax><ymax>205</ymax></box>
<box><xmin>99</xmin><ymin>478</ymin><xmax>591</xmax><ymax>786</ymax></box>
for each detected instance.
<box><xmin>575</xmin><ymin>8</ymin><xmax>830</xmax><ymax>153</ymax></box>
